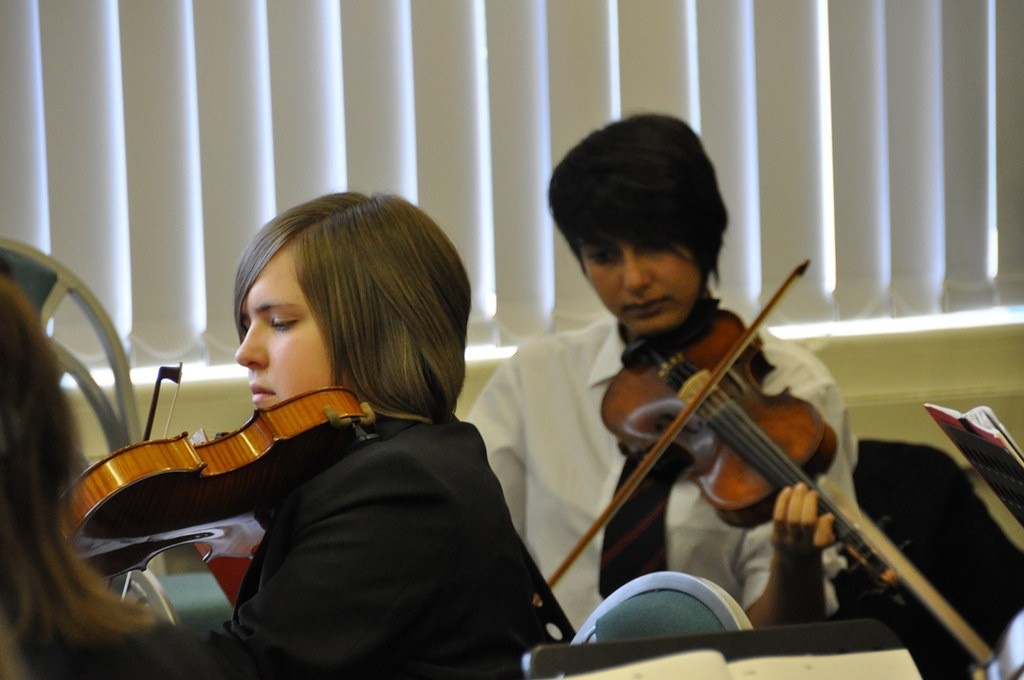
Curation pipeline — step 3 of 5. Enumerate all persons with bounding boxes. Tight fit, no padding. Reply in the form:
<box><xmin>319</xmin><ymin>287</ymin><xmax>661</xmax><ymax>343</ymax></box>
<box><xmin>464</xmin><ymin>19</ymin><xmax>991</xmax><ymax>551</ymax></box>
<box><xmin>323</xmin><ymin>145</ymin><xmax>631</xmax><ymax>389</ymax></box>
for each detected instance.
<box><xmin>469</xmin><ymin>110</ymin><xmax>859</xmax><ymax>629</ymax></box>
<box><xmin>0</xmin><ymin>275</ymin><xmax>254</xmax><ymax>680</ymax></box>
<box><xmin>200</xmin><ymin>191</ymin><xmax>546</xmax><ymax>680</ymax></box>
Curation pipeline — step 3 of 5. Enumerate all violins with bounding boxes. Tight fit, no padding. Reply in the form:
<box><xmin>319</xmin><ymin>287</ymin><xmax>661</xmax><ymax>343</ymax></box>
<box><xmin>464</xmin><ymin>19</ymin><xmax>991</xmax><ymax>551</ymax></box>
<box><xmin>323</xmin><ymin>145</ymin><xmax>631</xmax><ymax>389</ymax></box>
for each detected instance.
<box><xmin>598</xmin><ymin>308</ymin><xmax>913</xmax><ymax>601</ymax></box>
<box><xmin>54</xmin><ymin>387</ymin><xmax>365</xmax><ymax>583</ymax></box>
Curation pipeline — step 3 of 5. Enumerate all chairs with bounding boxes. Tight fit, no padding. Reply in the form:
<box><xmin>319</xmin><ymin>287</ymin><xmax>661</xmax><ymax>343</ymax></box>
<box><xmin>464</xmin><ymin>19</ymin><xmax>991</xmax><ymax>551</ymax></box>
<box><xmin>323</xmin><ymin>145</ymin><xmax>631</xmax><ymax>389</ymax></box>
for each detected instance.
<box><xmin>569</xmin><ymin>571</ymin><xmax>754</xmax><ymax>645</ymax></box>
